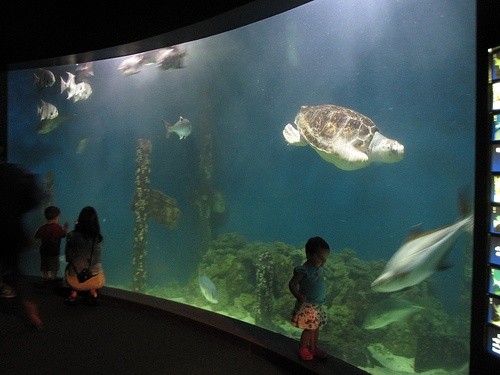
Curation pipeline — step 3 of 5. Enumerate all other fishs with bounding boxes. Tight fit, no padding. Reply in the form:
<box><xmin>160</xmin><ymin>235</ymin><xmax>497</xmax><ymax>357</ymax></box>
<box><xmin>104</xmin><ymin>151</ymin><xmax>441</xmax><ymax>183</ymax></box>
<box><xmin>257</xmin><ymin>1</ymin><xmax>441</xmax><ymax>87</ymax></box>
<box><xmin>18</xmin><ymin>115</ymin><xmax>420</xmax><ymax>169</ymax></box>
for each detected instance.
<box><xmin>361</xmin><ymin>215</ymin><xmax>474</xmax><ymax>331</ymax></box>
<box><xmin>132</xmin><ymin>191</ymin><xmax>184</xmax><ymax>231</ymax></box>
<box><xmin>161</xmin><ymin>116</ymin><xmax>192</xmax><ymax>141</ymax></box>
<box><xmin>32</xmin><ymin>42</ymin><xmax>187</xmax><ymax>135</ymax></box>
<box><xmin>198</xmin><ymin>273</ymin><xmax>219</xmax><ymax>304</ymax></box>
<box><xmin>75</xmin><ymin>137</ymin><xmax>89</xmax><ymax>154</ymax></box>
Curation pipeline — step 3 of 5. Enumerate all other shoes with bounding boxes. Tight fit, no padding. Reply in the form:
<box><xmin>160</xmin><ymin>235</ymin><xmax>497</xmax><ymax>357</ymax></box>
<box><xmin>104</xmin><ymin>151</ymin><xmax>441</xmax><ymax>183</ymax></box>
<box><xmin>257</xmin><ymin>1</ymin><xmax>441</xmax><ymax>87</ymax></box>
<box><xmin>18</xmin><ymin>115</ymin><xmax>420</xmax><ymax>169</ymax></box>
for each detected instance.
<box><xmin>312</xmin><ymin>347</ymin><xmax>328</xmax><ymax>360</ymax></box>
<box><xmin>297</xmin><ymin>345</ymin><xmax>314</xmax><ymax>361</ymax></box>
<box><xmin>0</xmin><ymin>283</ymin><xmax>16</xmax><ymax>298</ymax></box>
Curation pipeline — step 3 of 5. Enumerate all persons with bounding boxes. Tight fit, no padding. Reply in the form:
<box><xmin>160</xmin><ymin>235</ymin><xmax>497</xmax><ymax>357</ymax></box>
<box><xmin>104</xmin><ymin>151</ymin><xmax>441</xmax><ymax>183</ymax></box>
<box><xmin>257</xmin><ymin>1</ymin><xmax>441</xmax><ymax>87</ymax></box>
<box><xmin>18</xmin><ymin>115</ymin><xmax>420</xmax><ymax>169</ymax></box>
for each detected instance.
<box><xmin>35</xmin><ymin>206</ymin><xmax>68</xmax><ymax>282</ymax></box>
<box><xmin>64</xmin><ymin>206</ymin><xmax>104</xmax><ymax>301</ymax></box>
<box><xmin>0</xmin><ymin>263</ymin><xmax>43</xmax><ymax>330</ymax></box>
<box><xmin>289</xmin><ymin>236</ymin><xmax>331</xmax><ymax>361</ymax></box>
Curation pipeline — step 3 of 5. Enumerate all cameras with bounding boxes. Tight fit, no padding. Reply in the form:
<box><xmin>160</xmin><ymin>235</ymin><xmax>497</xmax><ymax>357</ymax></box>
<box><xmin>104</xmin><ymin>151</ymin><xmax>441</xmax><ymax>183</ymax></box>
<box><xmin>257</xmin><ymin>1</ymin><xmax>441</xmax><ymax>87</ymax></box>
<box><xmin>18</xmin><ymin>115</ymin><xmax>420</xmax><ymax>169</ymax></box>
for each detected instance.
<box><xmin>77</xmin><ymin>268</ymin><xmax>92</xmax><ymax>283</ymax></box>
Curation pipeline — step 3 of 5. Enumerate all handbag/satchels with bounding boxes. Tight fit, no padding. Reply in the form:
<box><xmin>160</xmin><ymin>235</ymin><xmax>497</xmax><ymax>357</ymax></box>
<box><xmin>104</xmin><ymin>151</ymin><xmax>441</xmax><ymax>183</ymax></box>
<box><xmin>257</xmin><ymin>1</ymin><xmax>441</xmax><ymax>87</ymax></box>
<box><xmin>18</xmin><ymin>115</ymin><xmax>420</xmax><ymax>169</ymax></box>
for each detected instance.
<box><xmin>78</xmin><ymin>269</ymin><xmax>92</xmax><ymax>283</ymax></box>
